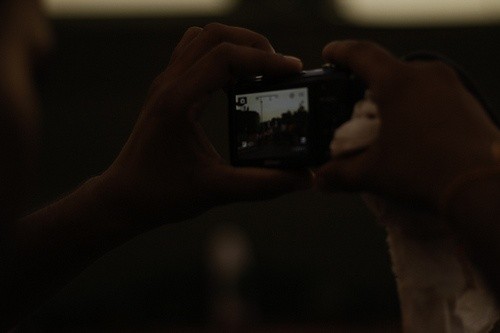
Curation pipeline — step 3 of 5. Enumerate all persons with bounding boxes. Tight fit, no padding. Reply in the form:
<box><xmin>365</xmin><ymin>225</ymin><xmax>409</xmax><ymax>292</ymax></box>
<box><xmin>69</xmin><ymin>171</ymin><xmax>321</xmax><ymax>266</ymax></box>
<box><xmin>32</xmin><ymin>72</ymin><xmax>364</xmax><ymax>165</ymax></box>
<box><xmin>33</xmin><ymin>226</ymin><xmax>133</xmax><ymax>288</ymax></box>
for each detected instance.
<box><xmin>0</xmin><ymin>17</ymin><xmax>500</xmax><ymax>332</ymax></box>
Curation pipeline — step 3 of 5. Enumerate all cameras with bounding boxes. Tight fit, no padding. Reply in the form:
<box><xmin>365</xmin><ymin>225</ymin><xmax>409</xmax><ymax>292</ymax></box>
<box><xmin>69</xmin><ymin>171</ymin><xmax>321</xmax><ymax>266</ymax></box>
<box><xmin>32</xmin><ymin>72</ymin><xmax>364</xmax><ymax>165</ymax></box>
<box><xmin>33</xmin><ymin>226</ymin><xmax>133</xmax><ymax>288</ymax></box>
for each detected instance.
<box><xmin>226</xmin><ymin>63</ymin><xmax>367</xmax><ymax>169</ymax></box>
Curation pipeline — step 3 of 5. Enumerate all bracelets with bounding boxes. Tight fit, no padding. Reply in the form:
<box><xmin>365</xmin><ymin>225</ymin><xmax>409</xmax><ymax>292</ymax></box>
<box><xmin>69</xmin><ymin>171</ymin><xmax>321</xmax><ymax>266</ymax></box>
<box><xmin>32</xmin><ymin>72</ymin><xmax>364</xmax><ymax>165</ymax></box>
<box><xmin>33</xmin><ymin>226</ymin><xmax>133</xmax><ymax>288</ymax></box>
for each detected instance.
<box><xmin>432</xmin><ymin>159</ymin><xmax>498</xmax><ymax>221</ymax></box>
